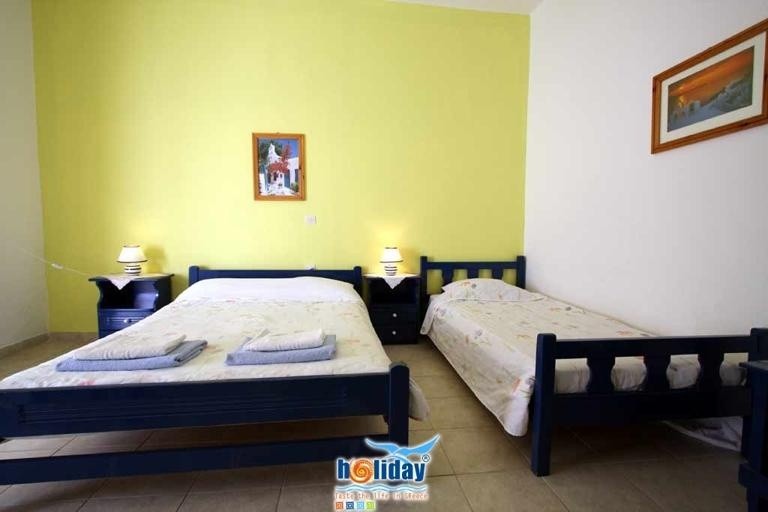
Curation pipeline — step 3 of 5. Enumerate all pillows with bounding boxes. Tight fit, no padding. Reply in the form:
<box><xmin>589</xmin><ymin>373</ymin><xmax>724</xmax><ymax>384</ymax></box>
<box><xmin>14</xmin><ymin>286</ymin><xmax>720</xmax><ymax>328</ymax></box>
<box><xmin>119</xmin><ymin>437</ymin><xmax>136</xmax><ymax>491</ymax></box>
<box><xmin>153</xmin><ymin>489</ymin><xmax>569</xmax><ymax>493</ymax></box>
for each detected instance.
<box><xmin>177</xmin><ymin>277</ymin><xmax>363</xmax><ymax>304</ymax></box>
<box><xmin>441</xmin><ymin>278</ymin><xmax>513</xmax><ymax>298</ymax></box>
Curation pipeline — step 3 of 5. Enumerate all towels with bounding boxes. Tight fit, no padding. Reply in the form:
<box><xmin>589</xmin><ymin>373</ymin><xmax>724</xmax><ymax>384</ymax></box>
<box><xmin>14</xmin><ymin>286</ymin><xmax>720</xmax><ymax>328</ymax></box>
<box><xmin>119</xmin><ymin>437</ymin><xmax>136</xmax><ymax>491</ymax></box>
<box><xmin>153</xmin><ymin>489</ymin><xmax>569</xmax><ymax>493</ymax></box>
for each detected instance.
<box><xmin>223</xmin><ymin>334</ymin><xmax>338</xmax><ymax>365</ymax></box>
<box><xmin>247</xmin><ymin>329</ymin><xmax>325</xmax><ymax>353</ymax></box>
<box><xmin>51</xmin><ymin>339</ymin><xmax>206</xmax><ymax>370</ymax></box>
<box><xmin>73</xmin><ymin>332</ymin><xmax>186</xmax><ymax>359</ymax></box>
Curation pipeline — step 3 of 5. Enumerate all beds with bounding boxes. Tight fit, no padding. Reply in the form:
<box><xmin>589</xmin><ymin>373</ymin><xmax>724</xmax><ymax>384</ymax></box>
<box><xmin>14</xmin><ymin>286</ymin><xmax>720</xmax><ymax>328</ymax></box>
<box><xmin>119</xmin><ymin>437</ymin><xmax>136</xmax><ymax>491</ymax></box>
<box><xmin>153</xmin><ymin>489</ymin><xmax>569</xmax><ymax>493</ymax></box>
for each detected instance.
<box><xmin>0</xmin><ymin>267</ymin><xmax>429</xmax><ymax>512</ymax></box>
<box><xmin>417</xmin><ymin>255</ymin><xmax>767</xmax><ymax>477</ymax></box>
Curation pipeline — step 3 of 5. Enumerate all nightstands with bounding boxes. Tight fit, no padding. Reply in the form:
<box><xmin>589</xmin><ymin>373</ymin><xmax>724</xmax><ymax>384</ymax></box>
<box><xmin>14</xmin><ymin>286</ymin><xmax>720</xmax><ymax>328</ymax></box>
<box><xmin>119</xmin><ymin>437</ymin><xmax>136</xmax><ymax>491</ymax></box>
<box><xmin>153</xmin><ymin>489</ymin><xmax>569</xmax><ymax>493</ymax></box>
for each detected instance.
<box><xmin>363</xmin><ymin>271</ymin><xmax>422</xmax><ymax>345</ymax></box>
<box><xmin>88</xmin><ymin>272</ymin><xmax>176</xmax><ymax>340</ymax></box>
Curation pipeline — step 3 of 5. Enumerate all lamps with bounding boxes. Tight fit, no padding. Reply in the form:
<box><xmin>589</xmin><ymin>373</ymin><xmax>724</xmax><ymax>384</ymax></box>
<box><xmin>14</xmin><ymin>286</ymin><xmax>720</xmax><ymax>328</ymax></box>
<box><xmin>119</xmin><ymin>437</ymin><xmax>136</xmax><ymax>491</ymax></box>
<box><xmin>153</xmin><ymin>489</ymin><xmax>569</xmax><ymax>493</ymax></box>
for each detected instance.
<box><xmin>118</xmin><ymin>244</ymin><xmax>147</xmax><ymax>275</ymax></box>
<box><xmin>380</xmin><ymin>247</ymin><xmax>403</xmax><ymax>276</ymax></box>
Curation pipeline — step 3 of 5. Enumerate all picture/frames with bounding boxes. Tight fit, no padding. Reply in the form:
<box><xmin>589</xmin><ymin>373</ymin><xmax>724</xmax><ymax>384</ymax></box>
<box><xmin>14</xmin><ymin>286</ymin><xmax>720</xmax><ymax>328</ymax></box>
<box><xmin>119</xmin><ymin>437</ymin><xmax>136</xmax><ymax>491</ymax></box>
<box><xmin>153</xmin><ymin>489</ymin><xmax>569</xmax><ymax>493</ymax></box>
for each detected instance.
<box><xmin>250</xmin><ymin>132</ymin><xmax>306</xmax><ymax>202</ymax></box>
<box><xmin>650</xmin><ymin>17</ymin><xmax>768</xmax><ymax>154</ymax></box>
